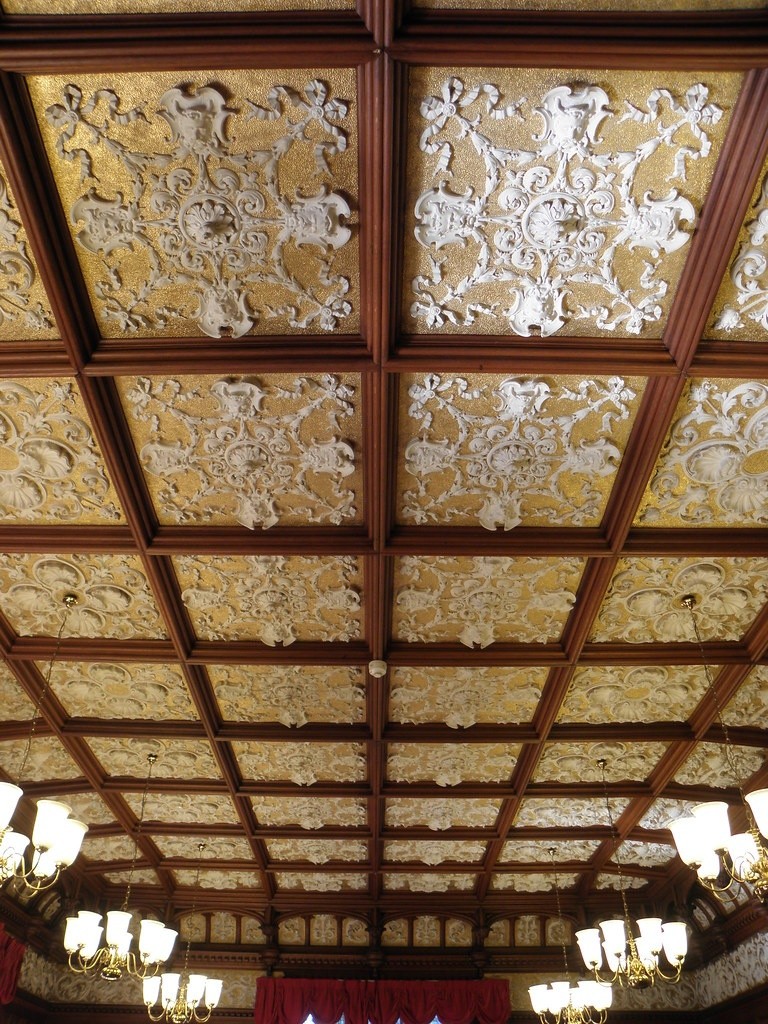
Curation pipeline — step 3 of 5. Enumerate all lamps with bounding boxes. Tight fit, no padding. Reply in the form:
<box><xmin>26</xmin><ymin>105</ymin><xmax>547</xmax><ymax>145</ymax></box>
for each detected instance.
<box><xmin>0</xmin><ymin>595</ymin><xmax>89</xmax><ymax>898</ymax></box>
<box><xmin>666</xmin><ymin>597</ymin><xmax>767</xmax><ymax>904</ymax></box>
<box><xmin>142</xmin><ymin>844</ymin><xmax>222</xmax><ymax>1023</ymax></box>
<box><xmin>527</xmin><ymin>848</ymin><xmax>612</xmax><ymax>1024</ymax></box>
<box><xmin>63</xmin><ymin>755</ymin><xmax>178</xmax><ymax>980</ymax></box>
<box><xmin>575</xmin><ymin>758</ymin><xmax>687</xmax><ymax>988</ymax></box>
